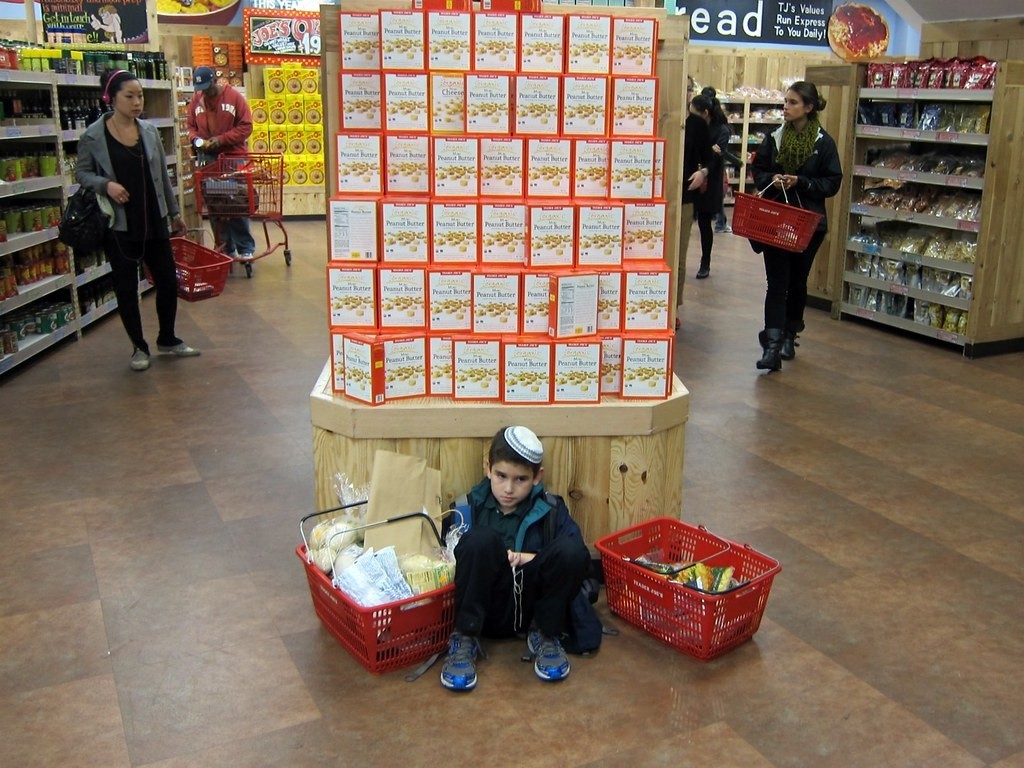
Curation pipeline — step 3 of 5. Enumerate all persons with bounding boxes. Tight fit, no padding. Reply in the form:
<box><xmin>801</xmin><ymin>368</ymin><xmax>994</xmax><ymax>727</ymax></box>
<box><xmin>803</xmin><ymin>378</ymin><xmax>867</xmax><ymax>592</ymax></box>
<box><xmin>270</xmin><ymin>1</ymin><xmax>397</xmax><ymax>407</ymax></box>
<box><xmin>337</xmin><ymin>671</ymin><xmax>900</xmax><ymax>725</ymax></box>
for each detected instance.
<box><xmin>187</xmin><ymin>67</ymin><xmax>256</xmax><ymax>263</ymax></box>
<box><xmin>74</xmin><ymin>68</ymin><xmax>201</xmax><ymax>370</ymax></box>
<box><xmin>747</xmin><ymin>81</ymin><xmax>843</xmax><ymax>370</ymax></box>
<box><xmin>440</xmin><ymin>424</ymin><xmax>591</xmax><ymax>690</ymax></box>
<box><xmin>675</xmin><ymin>73</ymin><xmax>733</xmax><ymax>329</ymax></box>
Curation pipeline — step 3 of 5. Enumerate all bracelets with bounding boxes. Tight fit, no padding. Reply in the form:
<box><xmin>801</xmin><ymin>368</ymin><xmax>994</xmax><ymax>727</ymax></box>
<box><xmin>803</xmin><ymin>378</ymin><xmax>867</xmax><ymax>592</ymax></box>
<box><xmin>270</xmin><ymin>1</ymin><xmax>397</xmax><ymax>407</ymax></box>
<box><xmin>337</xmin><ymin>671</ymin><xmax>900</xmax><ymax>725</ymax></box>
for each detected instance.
<box><xmin>700</xmin><ymin>169</ymin><xmax>706</xmax><ymax>178</ymax></box>
<box><xmin>170</xmin><ymin>214</ymin><xmax>181</xmax><ymax>221</ymax></box>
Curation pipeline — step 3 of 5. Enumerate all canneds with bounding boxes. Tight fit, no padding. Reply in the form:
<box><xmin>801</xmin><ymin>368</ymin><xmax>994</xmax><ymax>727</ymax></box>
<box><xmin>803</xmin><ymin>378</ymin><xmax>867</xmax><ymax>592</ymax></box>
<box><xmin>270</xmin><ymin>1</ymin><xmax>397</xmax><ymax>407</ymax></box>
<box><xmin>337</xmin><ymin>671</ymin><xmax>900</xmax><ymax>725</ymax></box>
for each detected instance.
<box><xmin>0</xmin><ymin>300</ymin><xmax>77</xmax><ymax>360</ymax></box>
<box><xmin>0</xmin><ymin>152</ymin><xmax>62</xmax><ymax>233</ymax></box>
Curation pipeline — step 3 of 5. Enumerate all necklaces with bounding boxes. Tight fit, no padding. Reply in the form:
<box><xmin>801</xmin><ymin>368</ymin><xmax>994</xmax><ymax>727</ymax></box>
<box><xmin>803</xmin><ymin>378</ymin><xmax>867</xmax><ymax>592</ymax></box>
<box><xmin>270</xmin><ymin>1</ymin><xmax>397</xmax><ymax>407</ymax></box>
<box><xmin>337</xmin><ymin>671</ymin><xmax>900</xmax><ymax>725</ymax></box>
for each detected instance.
<box><xmin>111</xmin><ymin>117</ymin><xmax>143</xmax><ymax>157</ymax></box>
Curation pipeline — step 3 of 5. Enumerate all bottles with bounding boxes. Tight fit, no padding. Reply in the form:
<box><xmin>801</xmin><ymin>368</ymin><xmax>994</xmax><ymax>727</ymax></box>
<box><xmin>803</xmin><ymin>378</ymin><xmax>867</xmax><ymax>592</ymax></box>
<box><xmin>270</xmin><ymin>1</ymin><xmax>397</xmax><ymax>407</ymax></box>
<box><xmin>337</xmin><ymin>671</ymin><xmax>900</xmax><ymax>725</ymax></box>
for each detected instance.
<box><xmin>0</xmin><ymin>89</ymin><xmax>52</xmax><ymax>118</ymax></box>
<box><xmin>90</xmin><ymin>51</ymin><xmax>169</xmax><ymax>81</ymax></box>
<box><xmin>59</xmin><ymin>91</ymin><xmax>113</xmax><ymax>130</ymax></box>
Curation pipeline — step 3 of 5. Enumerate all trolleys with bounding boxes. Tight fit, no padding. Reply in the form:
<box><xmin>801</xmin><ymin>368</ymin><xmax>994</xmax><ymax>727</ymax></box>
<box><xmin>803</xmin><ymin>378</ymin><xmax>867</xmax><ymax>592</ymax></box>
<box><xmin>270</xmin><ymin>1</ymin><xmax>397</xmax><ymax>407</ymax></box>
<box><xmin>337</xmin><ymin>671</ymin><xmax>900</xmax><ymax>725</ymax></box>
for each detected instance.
<box><xmin>194</xmin><ymin>150</ymin><xmax>291</xmax><ymax>278</ymax></box>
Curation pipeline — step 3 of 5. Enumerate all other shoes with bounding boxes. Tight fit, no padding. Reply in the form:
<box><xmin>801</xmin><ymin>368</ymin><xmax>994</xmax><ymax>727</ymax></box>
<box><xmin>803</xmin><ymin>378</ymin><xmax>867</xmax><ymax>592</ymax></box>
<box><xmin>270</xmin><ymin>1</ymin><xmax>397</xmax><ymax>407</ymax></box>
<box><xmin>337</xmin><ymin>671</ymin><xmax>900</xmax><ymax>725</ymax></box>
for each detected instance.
<box><xmin>229</xmin><ymin>248</ymin><xmax>239</xmax><ymax>260</ymax></box>
<box><xmin>675</xmin><ymin>318</ymin><xmax>681</xmax><ymax>329</ymax></box>
<box><xmin>240</xmin><ymin>251</ymin><xmax>253</xmax><ymax>258</ymax></box>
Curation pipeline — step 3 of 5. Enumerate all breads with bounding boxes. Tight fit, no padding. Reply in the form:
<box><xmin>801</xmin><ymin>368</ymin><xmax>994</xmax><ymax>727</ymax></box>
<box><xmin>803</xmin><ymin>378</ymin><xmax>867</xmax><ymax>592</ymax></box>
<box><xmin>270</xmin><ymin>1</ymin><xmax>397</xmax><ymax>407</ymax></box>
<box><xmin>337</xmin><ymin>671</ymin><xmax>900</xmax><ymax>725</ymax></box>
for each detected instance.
<box><xmin>401</xmin><ymin>554</ymin><xmax>447</xmax><ymax>573</ymax></box>
<box><xmin>306</xmin><ymin>508</ymin><xmax>365</xmax><ymax>574</ymax></box>
<box><xmin>715</xmin><ymin>86</ymin><xmax>787</xmax><ymax>120</ymax></box>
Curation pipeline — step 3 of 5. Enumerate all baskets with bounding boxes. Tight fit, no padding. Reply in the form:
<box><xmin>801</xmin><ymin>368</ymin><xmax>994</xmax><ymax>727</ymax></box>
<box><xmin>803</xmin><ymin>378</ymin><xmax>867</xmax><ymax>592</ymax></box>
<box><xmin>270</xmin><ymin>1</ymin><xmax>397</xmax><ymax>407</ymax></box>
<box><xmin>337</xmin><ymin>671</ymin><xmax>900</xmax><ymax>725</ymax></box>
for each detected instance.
<box><xmin>297</xmin><ymin>500</ymin><xmax>456</xmax><ymax>677</ymax></box>
<box><xmin>143</xmin><ymin>227</ymin><xmax>233</xmax><ymax>302</ymax></box>
<box><xmin>731</xmin><ymin>177</ymin><xmax>822</xmax><ymax>253</ymax></box>
<box><xmin>595</xmin><ymin>517</ymin><xmax>782</xmax><ymax>663</ymax></box>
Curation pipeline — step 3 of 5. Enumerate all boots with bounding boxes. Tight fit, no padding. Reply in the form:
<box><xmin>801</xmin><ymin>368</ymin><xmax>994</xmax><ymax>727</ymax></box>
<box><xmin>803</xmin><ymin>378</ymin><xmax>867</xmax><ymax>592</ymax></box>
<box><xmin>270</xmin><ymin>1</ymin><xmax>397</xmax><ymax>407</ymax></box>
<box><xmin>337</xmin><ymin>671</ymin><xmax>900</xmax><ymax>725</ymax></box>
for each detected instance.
<box><xmin>756</xmin><ymin>327</ymin><xmax>785</xmax><ymax>370</ymax></box>
<box><xmin>779</xmin><ymin>328</ymin><xmax>796</xmax><ymax>361</ymax></box>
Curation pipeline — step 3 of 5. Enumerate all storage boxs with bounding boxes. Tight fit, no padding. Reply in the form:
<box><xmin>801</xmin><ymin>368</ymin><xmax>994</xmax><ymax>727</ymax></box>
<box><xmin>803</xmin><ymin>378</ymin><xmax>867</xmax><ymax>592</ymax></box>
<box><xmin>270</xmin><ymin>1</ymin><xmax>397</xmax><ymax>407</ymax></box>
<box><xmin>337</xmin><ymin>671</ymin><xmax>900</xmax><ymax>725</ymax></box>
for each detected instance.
<box><xmin>247</xmin><ymin>8</ymin><xmax>676</xmax><ymax>406</ymax></box>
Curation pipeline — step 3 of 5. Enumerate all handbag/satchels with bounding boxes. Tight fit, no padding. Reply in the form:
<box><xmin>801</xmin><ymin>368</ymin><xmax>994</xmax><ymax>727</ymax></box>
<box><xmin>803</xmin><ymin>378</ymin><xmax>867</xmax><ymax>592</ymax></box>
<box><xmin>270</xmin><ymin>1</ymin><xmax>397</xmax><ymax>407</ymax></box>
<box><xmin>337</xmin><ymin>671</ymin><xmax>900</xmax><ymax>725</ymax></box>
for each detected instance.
<box><xmin>57</xmin><ymin>186</ymin><xmax>111</xmax><ymax>252</ymax></box>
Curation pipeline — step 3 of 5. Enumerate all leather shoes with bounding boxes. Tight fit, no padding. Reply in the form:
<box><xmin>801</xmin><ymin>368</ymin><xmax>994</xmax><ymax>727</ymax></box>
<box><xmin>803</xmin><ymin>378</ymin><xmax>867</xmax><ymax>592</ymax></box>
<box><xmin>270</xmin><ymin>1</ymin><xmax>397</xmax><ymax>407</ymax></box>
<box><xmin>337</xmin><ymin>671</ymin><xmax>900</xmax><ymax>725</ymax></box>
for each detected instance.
<box><xmin>696</xmin><ymin>264</ymin><xmax>710</xmax><ymax>278</ymax></box>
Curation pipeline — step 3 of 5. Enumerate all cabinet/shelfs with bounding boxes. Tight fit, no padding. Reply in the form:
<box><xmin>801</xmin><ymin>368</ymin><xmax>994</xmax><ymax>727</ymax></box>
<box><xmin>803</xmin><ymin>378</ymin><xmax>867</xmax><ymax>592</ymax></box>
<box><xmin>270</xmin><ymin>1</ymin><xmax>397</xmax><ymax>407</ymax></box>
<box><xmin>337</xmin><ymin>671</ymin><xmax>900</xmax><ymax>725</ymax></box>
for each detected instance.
<box><xmin>0</xmin><ymin>61</ymin><xmax>207</xmax><ymax>385</ymax></box>
<box><xmin>829</xmin><ymin>63</ymin><xmax>1024</xmax><ymax>358</ymax></box>
<box><xmin>685</xmin><ymin>90</ymin><xmax>812</xmax><ymax>204</ymax></box>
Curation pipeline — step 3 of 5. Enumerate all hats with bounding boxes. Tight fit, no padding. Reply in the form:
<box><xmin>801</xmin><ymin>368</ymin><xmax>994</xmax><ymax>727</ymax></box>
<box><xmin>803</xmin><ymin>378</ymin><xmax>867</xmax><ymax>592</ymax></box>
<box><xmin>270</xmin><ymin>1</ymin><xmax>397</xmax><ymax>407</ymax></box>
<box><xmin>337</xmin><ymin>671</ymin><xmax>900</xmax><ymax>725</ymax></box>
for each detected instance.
<box><xmin>193</xmin><ymin>66</ymin><xmax>216</xmax><ymax>91</ymax></box>
<box><xmin>504</xmin><ymin>425</ymin><xmax>543</xmax><ymax>464</ymax></box>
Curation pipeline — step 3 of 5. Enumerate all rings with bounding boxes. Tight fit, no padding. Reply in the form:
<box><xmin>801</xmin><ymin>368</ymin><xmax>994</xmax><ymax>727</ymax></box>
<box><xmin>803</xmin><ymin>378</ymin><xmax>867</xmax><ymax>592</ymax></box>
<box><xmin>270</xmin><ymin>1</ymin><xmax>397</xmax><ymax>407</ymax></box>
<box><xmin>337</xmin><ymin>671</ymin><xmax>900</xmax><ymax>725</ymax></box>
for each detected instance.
<box><xmin>119</xmin><ymin>197</ymin><xmax>123</xmax><ymax>201</ymax></box>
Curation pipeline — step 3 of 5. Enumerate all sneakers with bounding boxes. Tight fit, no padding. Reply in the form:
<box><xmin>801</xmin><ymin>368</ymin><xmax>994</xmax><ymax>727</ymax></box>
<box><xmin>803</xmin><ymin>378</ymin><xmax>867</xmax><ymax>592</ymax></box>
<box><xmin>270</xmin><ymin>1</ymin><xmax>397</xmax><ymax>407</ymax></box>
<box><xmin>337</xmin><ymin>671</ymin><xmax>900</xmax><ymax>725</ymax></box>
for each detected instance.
<box><xmin>714</xmin><ymin>223</ymin><xmax>732</xmax><ymax>233</ymax></box>
<box><xmin>527</xmin><ymin>628</ymin><xmax>570</xmax><ymax>681</ymax></box>
<box><xmin>440</xmin><ymin>632</ymin><xmax>487</xmax><ymax>691</ymax></box>
<box><xmin>132</xmin><ymin>348</ymin><xmax>149</xmax><ymax>370</ymax></box>
<box><xmin>159</xmin><ymin>341</ymin><xmax>201</xmax><ymax>356</ymax></box>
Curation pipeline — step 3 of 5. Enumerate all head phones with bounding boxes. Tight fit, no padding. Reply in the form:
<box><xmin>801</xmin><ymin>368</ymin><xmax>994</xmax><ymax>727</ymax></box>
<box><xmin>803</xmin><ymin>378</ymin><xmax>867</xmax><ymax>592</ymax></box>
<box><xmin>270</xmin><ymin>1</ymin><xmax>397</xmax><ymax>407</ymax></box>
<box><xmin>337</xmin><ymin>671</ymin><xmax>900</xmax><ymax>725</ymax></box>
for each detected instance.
<box><xmin>102</xmin><ymin>70</ymin><xmax>130</xmax><ymax>104</ymax></box>
